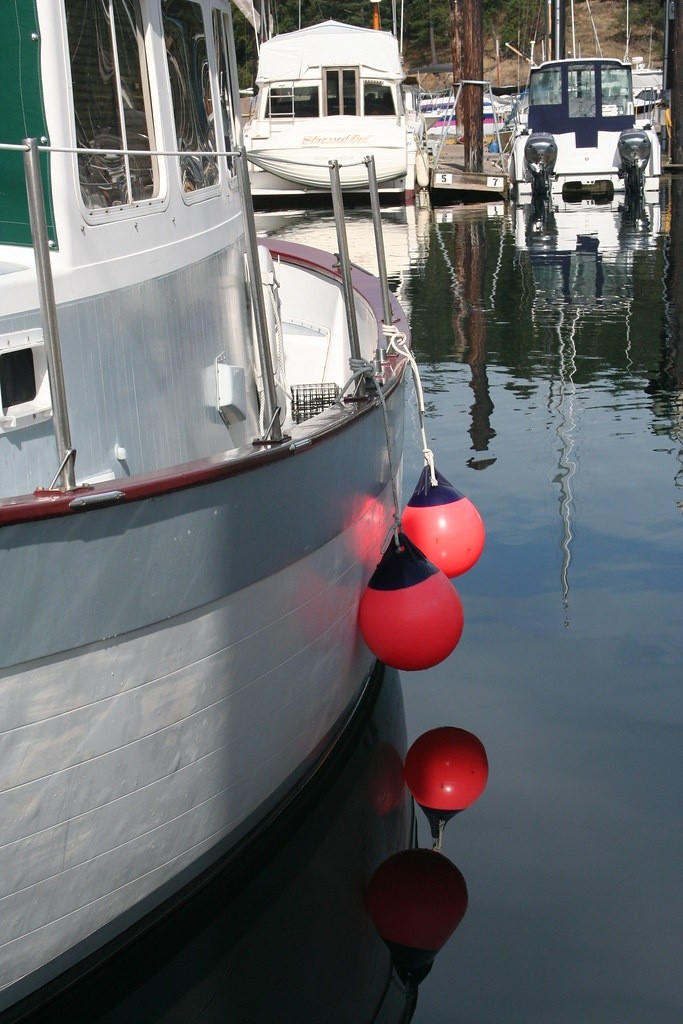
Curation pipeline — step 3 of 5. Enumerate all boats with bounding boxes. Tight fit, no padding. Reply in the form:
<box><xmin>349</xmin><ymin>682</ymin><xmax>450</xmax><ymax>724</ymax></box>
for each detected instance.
<box><xmin>1</xmin><ymin>0</ymin><xmax>419</xmax><ymax>1024</ymax></box>
<box><xmin>240</xmin><ymin>18</ymin><xmax>428</xmax><ymax>210</ymax></box>
<box><xmin>504</xmin><ymin>53</ymin><xmax>662</xmax><ymax>200</ymax></box>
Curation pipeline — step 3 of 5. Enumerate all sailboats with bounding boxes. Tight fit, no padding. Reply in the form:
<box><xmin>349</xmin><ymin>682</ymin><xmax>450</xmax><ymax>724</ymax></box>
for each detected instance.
<box><xmin>394</xmin><ymin>1</ymin><xmax>671</xmax><ymax>138</ymax></box>
